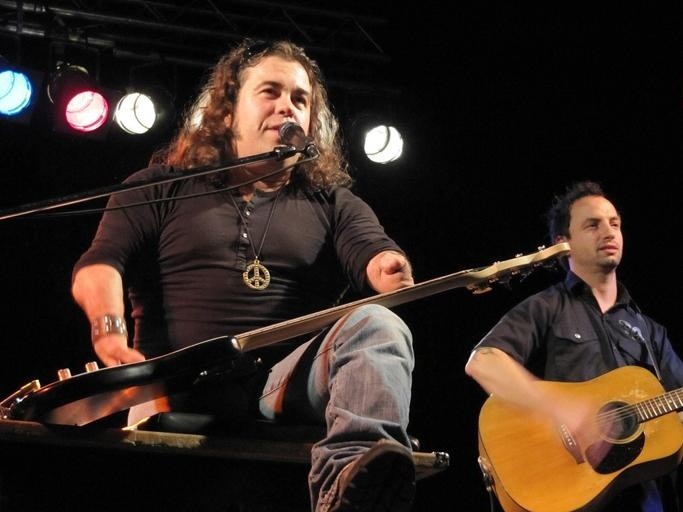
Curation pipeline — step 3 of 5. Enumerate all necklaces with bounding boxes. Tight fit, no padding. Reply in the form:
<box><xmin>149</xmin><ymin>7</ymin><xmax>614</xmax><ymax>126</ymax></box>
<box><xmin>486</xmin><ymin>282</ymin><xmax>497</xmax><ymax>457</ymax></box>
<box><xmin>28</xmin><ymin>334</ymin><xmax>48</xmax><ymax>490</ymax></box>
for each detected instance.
<box><xmin>216</xmin><ymin>174</ymin><xmax>290</xmax><ymax>291</ymax></box>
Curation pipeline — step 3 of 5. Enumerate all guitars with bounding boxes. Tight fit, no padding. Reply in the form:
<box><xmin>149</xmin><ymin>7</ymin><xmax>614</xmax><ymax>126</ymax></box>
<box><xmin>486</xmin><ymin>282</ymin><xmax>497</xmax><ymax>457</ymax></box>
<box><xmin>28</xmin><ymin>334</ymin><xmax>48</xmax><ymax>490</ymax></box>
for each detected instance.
<box><xmin>477</xmin><ymin>364</ymin><xmax>682</xmax><ymax>508</ymax></box>
<box><xmin>9</xmin><ymin>241</ymin><xmax>571</xmax><ymax>423</ymax></box>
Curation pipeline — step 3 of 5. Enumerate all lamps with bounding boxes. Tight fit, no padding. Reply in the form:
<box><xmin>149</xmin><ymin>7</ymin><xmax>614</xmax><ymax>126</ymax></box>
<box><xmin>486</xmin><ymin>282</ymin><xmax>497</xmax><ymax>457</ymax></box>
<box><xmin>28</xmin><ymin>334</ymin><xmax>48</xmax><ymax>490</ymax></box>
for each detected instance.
<box><xmin>43</xmin><ymin>2</ymin><xmax>178</xmax><ymax>142</ymax></box>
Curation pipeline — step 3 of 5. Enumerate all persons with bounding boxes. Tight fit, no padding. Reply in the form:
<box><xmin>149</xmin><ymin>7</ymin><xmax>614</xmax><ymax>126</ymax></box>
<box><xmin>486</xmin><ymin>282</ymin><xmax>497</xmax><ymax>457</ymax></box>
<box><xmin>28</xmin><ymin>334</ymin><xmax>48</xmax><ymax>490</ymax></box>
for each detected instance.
<box><xmin>67</xmin><ymin>37</ymin><xmax>417</xmax><ymax>512</ymax></box>
<box><xmin>463</xmin><ymin>177</ymin><xmax>683</xmax><ymax>512</ymax></box>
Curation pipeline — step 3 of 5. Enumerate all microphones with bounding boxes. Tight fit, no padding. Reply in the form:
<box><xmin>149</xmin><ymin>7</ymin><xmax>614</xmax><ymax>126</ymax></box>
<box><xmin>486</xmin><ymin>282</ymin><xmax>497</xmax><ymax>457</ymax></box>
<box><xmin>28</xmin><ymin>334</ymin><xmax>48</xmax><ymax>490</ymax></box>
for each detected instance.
<box><xmin>278</xmin><ymin>121</ymin><xmax>319</xmax><ymax>159</ymax></box>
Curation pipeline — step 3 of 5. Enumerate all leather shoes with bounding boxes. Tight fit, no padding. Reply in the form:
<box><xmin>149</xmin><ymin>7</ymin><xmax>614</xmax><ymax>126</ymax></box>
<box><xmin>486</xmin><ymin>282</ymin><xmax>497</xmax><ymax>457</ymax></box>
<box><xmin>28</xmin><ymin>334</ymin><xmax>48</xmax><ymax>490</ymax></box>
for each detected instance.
<box><xmin>326</xmin><ymin>439</ymin><xmax>415</xmax><ymax>512</ymax></box>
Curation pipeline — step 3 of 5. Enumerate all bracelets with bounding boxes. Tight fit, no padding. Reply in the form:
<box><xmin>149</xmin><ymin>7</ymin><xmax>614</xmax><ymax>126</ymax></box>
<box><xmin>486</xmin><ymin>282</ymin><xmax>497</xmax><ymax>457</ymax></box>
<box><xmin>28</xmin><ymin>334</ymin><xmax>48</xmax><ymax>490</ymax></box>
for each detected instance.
<box><xmin>89</xmin><ymin>314</ymin><xmax>129</xmax><ymax>338</ymax></box>
